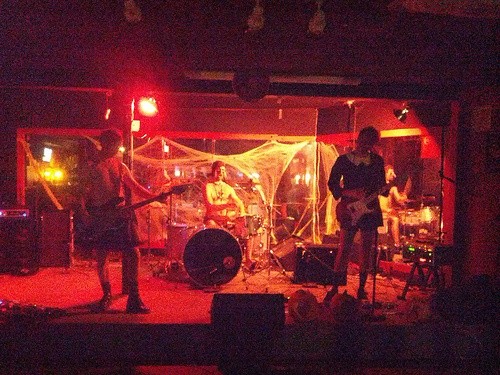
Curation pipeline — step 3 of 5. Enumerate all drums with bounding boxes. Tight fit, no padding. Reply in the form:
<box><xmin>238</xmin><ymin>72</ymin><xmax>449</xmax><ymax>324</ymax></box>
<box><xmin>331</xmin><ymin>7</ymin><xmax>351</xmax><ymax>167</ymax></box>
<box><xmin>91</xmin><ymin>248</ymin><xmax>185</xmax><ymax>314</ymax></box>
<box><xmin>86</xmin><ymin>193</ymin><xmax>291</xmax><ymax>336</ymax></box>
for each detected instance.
<box><xmin>164</xmin><ymin>223</ymin><xmax>206</xmax><ymax>259</ymax></box>
<box><xmin>175</xmin><ymin>225</ymin><xmax>246</xmax><ymax>288</ymax></box>
<box><xmin>230</xmin><ymin>213</ymin><xmax>263</xmax><ymax>241</ymax></box>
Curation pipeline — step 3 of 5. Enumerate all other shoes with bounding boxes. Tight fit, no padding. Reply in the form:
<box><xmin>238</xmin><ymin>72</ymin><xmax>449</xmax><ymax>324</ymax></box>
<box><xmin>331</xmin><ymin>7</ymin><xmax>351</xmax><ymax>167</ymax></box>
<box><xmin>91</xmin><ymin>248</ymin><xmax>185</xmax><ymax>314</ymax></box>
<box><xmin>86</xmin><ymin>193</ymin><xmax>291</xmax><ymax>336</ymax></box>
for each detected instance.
<box><xmin>125</xmin><ymin>299</ymin><xmax>147</xmax><ymax>314</ymax></box>
<box><xmin>357</xmin><ymin>289</ymin><xmax>368</xmax><ymax>300</ymax></box>
<box><xmin>95</xmin><ymin>295</ymin><xmax>111</xmax><ymax>309</ymax></box>
<box><xmin>324</xmin><ymin>285</ymin><xmax>339</xmax><ymax>301</ymax></box>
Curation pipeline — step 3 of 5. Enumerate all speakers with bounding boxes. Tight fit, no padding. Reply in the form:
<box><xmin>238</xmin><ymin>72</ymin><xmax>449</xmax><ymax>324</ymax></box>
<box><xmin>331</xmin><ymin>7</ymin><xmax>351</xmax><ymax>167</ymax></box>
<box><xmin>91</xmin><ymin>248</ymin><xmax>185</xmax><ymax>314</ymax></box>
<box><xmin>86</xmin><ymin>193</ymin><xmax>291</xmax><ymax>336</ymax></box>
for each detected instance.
<box><xmin>0</xmin><ymin>217</ymin><xmax>41</xmax><ymax>274</ymax></box>
<box><xmin>210</xmin><ymin>294</ymin><xmax>285</xmax><ymax>327</ymax></box>
<box><xmin>294</xmin><ymin>244</ymin><xmax>347</xmax><ymax>285</ymax></box>
<box><xmin>37</xmin><ymin>208</ymin><xmax>73</xmax><ymax>267</ymax></box>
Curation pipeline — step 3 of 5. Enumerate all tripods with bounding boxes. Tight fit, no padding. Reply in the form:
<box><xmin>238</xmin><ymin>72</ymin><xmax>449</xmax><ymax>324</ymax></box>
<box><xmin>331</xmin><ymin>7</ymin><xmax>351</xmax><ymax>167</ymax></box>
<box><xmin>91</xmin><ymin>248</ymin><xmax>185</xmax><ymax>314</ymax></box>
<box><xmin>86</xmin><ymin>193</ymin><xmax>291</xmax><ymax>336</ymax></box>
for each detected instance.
<box><xmin>147</xmin><ymin>178</ymin><xmax>184</xmax><ymax>278</ymax></box>
<box><xmin>355</xmin><ymin>150</ymin><xmax>386</xmax><ymax>326</ymax></box>
<box><xmin>241</xmin><ymin>203</ymin><xmax>294</xmax><ymax>294</ymax></box>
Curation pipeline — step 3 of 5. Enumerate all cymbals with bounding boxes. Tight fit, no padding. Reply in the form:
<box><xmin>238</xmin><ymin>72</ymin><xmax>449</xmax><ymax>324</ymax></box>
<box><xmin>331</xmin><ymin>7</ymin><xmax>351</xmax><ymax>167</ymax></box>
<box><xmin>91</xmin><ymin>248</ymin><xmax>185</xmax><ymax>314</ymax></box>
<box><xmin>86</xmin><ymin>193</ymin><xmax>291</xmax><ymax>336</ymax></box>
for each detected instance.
<box><xmin>265</xmin><ymin>204</ymin><xmax>282</xmax><ymax>207</ymax></box>
<box><xmin>284</xmin><ymin>201</ymin><xmax>302</xmax><ymax>205</ymax></box>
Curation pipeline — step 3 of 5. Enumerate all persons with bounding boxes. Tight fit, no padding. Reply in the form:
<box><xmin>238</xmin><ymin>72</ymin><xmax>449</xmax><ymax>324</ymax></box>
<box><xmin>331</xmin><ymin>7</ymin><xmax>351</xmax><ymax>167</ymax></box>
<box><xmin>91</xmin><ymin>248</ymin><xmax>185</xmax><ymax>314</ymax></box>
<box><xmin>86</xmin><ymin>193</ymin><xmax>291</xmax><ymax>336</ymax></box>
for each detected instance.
<box><xmin>325</xmin><ymin>127</ymin><xmax>395</xmax><ymax>300</ymax></box>
<box><xmin>380</xmin><ymin>165</ymin><xmax>412</xmax><ymax>247</ymax></box>
<box><xmin>79</xmin><ymin>128</ymin><xmax>149</xmax><ymax>313</ymax></box>
<box><xmin>276</xmin><ymin>167</ymin><xmax>290</xmax><ymax>217</ymax></box>
<box><xmin>205</xmin><ymin>161</ymin><xmax>255</xmax><ymax>269</ymax></box>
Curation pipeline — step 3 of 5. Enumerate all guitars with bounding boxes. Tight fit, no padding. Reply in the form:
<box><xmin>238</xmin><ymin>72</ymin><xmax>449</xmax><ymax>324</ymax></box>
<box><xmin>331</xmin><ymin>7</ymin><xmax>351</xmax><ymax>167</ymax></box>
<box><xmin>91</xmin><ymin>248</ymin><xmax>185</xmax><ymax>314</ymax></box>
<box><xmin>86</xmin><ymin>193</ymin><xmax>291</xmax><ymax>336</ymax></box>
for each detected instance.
<box><xmin>73</xmin><ymin>183</ymin><xmax>193</xmax><ymax>245</ymax></box>
<box><xmin>334</xmin><ymin>175</ymin><xmax>403</xmax><ymax>234</ymax></box>
